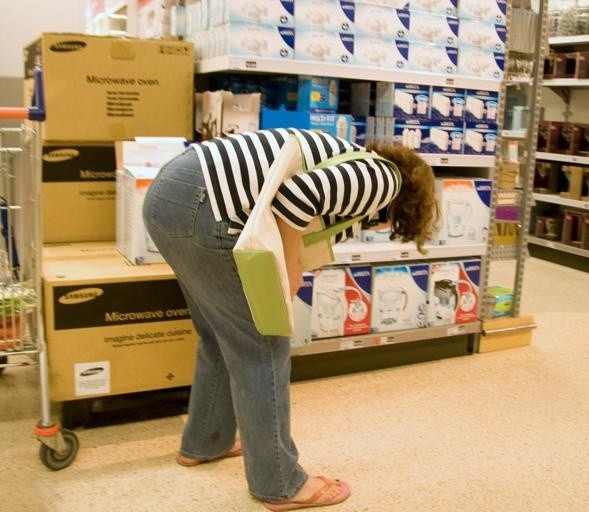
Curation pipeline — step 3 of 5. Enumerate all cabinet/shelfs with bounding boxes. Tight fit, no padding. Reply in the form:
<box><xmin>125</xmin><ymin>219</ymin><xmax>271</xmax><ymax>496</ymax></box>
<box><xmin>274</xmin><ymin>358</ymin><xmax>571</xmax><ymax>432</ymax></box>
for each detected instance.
<box><xmin>201</xmin><ymin>57</ymin><xmax>503</xmax><ymax>355</ymax></box>
<box><xmin>522</xmin><ymin>31</ymin><xmax>589</xmax><ymax>254</ymax></box>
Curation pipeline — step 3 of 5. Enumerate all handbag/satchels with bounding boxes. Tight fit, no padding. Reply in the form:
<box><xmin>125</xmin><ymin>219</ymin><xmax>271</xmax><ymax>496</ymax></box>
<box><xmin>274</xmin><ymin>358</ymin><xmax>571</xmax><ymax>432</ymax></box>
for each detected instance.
<box><xmin>231</xmin><ymin>132</ymin><xmax>403</xmax><ymax>338</ymax></box>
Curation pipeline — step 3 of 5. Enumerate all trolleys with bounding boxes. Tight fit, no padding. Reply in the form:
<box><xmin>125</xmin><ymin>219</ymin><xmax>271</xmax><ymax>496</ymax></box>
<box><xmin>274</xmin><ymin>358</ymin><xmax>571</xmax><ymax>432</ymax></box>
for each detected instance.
<box><xmin>1</xmin><ymin>107</ymin><xmax>79</xmax><ymax>471</ymax></box>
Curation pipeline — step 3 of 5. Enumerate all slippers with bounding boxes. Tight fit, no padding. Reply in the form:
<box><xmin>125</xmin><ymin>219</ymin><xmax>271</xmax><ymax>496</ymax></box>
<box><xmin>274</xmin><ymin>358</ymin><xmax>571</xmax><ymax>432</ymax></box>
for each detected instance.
<box><xmin>175</xmin><ymin>436</ymin><xmax>244</xmax><ymax>468</ymax></box>
<box><xmin>264</xmin><ymin>475</ymin><xmax>351</xmax><ymax>512</ymax></box>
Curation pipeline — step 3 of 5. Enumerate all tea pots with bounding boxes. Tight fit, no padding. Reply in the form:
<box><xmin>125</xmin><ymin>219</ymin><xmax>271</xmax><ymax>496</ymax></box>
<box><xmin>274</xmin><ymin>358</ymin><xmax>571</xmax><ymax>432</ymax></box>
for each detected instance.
<box><xmin>317</xmin><ymin>280</ymin><xmax>458</xmax><ymax>333</ymax></box>
<box><xmin>447</xmin><ymin>199</ymin><xmax>473</xmax><ymax>236</ymax></box>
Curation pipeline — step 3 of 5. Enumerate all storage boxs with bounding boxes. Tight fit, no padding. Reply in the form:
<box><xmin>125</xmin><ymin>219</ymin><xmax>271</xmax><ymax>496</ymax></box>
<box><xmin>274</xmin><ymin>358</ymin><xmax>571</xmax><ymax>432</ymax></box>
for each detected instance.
<box><xmin>32</xmin><ymin>140</ymin><xmax>205</xmax><ymax>244</ymax></box>
<box><xmin>38</xmin><ymin>246</ymin><xmax>204</xmax><ymax>404</ymax></box>
<box><xmin>117</xmin><ymin>135</ymin><xmax>186</xmax><ymax>267</ymax></box>
<box><xmin>20</xmin><ymin>34</ymin><xmax>204</xmax><ymax>143</ymax></box>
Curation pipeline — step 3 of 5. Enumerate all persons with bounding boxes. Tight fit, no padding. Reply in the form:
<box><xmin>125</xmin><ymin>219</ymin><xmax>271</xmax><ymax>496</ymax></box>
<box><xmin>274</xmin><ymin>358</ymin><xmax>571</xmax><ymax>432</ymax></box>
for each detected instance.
<box><xmin>142</xmin><ymin>125</ymin><xmax>443</xmax><ymax>512</ymax></box>
